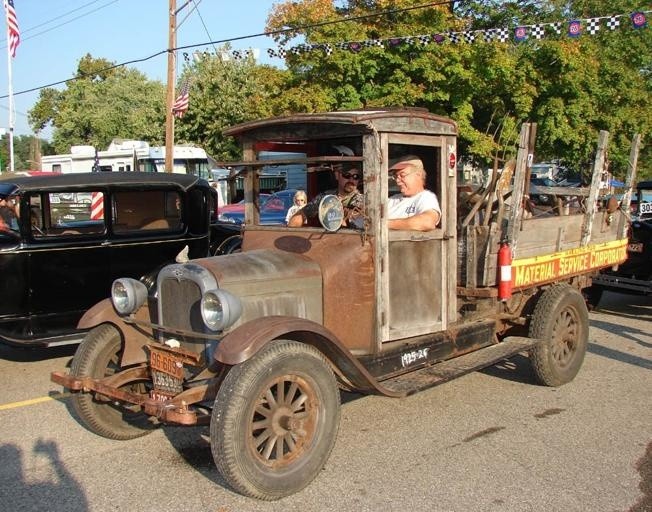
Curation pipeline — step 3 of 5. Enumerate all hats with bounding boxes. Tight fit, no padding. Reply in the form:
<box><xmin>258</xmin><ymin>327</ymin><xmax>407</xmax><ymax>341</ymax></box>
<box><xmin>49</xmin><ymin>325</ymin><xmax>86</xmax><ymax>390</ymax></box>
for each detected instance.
<box><xmin>388</xmin><ymin>154</ymin><xmax>425</xmax><ymax>172</ymax></box>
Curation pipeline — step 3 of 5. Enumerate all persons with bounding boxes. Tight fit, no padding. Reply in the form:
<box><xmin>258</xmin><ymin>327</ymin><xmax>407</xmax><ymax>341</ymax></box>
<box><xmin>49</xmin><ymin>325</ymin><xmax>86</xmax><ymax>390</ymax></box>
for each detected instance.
<box><xmin>385</xmin><ymin>153</ymin><xmax>442</xmax><ymax>232</ymax></box>
<box><xmin>285</xmin><ymin>160</ymin><xmax>367</xmax><ymax>233</ymax></box>
<box><xmin>285</xmin><ymin>190</ymin><xmax>308</xmax><ymax>227</ymax></box>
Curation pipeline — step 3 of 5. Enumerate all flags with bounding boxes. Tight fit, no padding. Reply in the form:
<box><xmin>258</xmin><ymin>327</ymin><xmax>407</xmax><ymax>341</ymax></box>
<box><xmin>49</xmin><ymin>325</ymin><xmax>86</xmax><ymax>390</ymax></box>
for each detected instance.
<box><xmin>89</xmin><ymin>155</ymin><xmax>107</xmax><ymax>222</ymax></box>
<box><xmin>3</xmin><ymin>0</ymin><xmax>21</xmax><ymax>59</ymax></box>
<box><xmin>182</xmin><ymin>11</ymin><xmax>646</xmax><ymax>63</ymax></box>
<box><xmin>168</xmin><ymin>79</ymin><xmax>191</xmax><ymax>119</ymax></box>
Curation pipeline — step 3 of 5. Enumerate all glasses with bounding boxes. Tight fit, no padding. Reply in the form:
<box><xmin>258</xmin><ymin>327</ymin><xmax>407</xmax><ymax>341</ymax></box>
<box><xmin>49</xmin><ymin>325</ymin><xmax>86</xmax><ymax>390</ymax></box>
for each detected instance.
<box><xmin>391</xmin><ymin>172</ymin><xmax>412</xmax><ymax>181</ymax></box>
<box><xmin>339</xmin><ymin>172</ymin><xmax>362</xmax><ymax>180</ymax></box>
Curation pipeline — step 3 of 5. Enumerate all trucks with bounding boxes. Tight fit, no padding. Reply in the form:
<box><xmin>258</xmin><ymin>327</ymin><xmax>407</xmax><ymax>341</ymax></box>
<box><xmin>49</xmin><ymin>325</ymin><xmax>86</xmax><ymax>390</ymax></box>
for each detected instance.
<box><xmin>50</xmin><ymin>106</ymin><xmax>640</xmax><ymax>501</ymax></box>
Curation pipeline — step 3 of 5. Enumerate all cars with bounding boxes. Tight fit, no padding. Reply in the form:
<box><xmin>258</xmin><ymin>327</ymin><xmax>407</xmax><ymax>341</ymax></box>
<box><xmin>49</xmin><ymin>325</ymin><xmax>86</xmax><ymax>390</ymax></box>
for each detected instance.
<box><xmin>530</xmin><ymin>159</ymin><xmax>652</xmax><ymax>307</ymax></box>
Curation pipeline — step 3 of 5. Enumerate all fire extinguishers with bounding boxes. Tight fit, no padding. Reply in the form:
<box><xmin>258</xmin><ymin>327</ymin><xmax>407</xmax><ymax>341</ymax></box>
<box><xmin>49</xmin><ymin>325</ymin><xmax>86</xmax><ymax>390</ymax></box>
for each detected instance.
<box><xmin>498</xmin><ymin>237</ymin><xmax>511</xmax><ymax>300</ymax></box>
